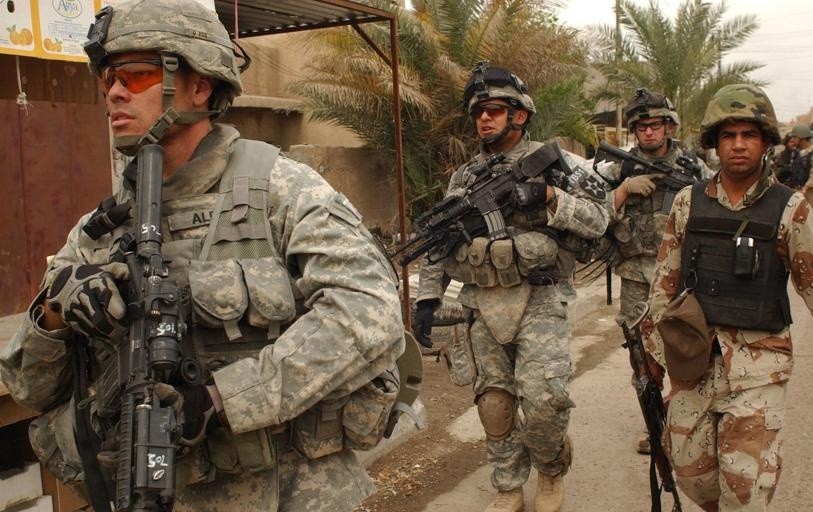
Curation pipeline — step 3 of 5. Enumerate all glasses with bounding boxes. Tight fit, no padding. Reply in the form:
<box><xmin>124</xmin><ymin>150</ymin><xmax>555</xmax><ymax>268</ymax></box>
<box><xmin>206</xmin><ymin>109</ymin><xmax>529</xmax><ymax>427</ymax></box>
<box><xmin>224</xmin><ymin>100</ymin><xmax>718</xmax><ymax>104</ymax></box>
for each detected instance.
<box><xmin>103</xmin><ymin>59</ymin><xmax>163</xmax><ymax>94</ymax></box>
<box><xmin>633</xmin><ymin>120</ymin><xmax>664</xmax><ymax>131</ymax></box>
<box><xmin>471</xmin><ymin>104</ymin><xmax>510</xmax><ymax>118</ymax></box>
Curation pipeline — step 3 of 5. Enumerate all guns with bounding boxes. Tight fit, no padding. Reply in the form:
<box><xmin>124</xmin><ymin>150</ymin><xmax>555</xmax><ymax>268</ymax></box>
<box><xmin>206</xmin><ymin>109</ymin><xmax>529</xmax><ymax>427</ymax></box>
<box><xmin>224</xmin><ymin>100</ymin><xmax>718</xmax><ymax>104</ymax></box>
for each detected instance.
<box><xmin>389</xmin><ymin>142</ymin><xmax>572</xmax><ymax>267</ymax></box>
<box><xmin>615</xmin><ymin>301</ymin><xmax>682</xmax><ymax>512</ymax></box>
<box><xmin>69</xmin><ymin>144</ymin><xmax>201</xmax><ymax>511</ymax></box>
<box><xmin>593</xmin><ymin>139</ymin><xmax>701</xmax><ymax>193</ymax></box>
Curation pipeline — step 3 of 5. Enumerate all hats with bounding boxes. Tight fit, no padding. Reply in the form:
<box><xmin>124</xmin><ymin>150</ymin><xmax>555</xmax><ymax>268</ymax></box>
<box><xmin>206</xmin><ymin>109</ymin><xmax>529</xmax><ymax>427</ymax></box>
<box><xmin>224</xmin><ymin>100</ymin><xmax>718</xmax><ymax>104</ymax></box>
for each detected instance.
<box><xmin>658</xmin><ymin>289</ymin><xmax>710</xmax><ymax>382</ymax></box>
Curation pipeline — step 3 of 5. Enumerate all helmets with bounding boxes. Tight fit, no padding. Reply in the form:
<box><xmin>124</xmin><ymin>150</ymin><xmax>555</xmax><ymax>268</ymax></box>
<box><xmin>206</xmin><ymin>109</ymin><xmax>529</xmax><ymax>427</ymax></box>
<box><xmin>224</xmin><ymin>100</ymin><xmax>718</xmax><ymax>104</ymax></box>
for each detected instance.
<box><xmin>699</xmin><ymin>84</ymin><xmax>781</xmax><ymax>149</ymax></box>
<box><xmin>464</xmin><ymin>59</ymin><xmax>536</xmax><ymax>123</ymax></box>
<box><xmin>783</xmin><ymin>124</ymin><xmax>813</xmax><ymax>145</ymax></box>
<box><xmin>624</xmin><ymin>88</ymin><xmax>680</xmax><ymax>132</ymax></box>
<box><xmin>82</xmin><ymin>0</ymin><xmax>243</xmax><ymax>95</ymax></box>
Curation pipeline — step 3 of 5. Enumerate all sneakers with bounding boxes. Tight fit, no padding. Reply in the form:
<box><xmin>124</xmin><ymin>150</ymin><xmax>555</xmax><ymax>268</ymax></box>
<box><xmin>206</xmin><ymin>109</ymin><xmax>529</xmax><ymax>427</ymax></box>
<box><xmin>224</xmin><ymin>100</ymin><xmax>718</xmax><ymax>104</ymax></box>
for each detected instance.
<box><xmin>636</xmin><ymin>430</ymin><xmax>652</xmax><ymax>453</ymax></box>
<box><xmin>534</xmin><ymin>472</ymin><xmax>565</xmax><ymax>512</ymax></box>
<box><xmin>483</xmin><ymin>485</ymin><xmax>523</xmax><ymax>512</ymax></box>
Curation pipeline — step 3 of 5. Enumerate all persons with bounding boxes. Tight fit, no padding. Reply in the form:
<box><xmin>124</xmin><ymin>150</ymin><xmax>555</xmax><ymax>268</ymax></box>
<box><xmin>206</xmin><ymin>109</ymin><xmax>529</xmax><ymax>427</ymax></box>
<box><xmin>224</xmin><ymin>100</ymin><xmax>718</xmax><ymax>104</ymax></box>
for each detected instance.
<box><xmin>771</xmin><ymin>132</ymin><xmax>801</xmax><ymax>183</ymax></box>
<box><xmin>786</xmin><ymin>126</ymin><xmax>812</xmax><ymax>195</ymax></box>
<box><xmin>0</xmin><ymin>0</ymin><xmax>406</xmax><ymax>511</ymax></box>
<box><xmin>637</xmin><ymin>83</ymin><xmax>811</xmax><ymax>512</ymax></box>
<box><xmin>604</xmin><ymin>87</ymin><xmax>717</xmax><ymax>453</ymax></box>
<box><xmin>410</xmin><ymin>62</ymin><xmax>611</xmax><ymax>511</ymax></box>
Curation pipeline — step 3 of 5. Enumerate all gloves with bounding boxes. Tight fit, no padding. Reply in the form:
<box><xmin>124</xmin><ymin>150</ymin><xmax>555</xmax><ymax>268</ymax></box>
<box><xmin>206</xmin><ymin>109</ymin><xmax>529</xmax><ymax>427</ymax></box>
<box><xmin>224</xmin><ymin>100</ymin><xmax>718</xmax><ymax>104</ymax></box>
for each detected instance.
<box><xmin>47</xmin><ymin>262</ymin><xmax>130</xmax><ymax>344</ymax></box>
<box><xmin>509</xmin><ymin>182</ymin><xmax>546</xmax><ymax>212</ymax></box>
<box><xmin>413</xmin><ymin>301</ymin><xmax>436</xmax><ymax>348</ymax></box>
<box><xmin>623</xmin><ymin>173</ymin><xmax>666</xmax><ymax>197</ymax></box>
<box><xmin>96</xmin><ymin>383</ymin><xmax>220</xmax><ymax>468</ymax></box>
